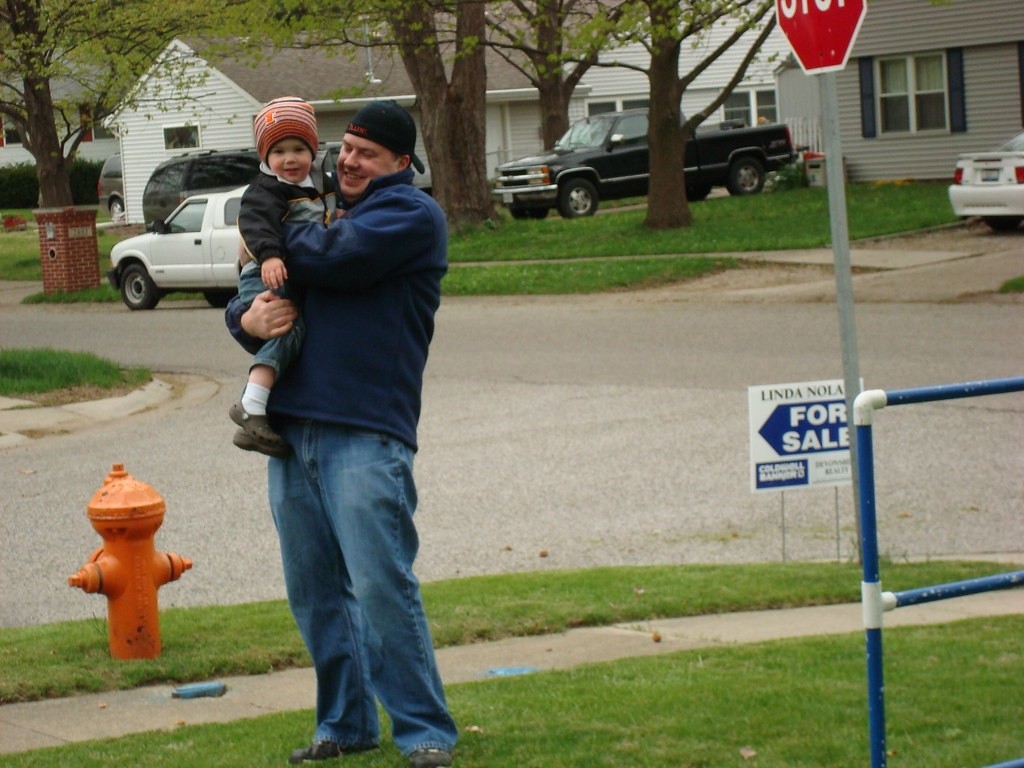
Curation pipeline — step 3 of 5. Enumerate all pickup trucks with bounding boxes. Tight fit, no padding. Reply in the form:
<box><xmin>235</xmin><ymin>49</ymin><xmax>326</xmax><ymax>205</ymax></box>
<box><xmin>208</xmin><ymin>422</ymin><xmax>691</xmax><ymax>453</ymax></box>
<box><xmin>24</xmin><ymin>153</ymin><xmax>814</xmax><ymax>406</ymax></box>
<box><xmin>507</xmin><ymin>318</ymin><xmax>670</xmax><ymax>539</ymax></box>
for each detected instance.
<box><xmin>106</xmin><ymin>192</ymin><xmax>242</xmax><ymax>310</ymax></box>
<box><xmin>490</xmin><ymin>108</ymin><xmax>796</xmax><ymax>219</ymax></box>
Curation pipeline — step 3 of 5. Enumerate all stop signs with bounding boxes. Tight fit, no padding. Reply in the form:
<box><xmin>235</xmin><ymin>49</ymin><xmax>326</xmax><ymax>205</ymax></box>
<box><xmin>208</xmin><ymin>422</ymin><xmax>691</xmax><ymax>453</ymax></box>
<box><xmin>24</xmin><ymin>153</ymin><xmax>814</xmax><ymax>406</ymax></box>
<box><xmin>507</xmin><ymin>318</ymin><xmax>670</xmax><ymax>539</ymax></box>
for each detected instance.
<box><xmin>775</xmin><ymin>0</ymin><xmax>866</xmax><ymax>75</ymax></box>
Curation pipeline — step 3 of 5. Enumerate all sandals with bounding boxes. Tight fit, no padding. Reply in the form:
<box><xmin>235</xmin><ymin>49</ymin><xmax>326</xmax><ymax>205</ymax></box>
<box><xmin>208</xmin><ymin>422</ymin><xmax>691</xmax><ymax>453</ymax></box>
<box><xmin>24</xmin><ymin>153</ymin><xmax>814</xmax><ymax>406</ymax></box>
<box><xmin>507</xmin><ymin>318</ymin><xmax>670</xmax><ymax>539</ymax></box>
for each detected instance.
<box><xmin>229</xmin><ymin>399</ymin><xmax>294</xmax><ymax>458</ymax></box>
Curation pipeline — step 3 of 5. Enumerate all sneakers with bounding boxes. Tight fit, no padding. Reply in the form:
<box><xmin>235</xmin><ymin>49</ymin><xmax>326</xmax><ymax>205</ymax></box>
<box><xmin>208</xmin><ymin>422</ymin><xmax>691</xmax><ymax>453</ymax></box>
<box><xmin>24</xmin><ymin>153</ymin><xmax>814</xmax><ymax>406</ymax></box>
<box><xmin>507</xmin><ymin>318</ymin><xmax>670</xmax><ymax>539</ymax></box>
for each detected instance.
<box><xmin>409</xmin><ymin>748</ymin><xmax>452</xmax><ymax>768</ymax></box>
<box><xmin>289</xmin><ymin>736</ymin><xmax>378</xmax><ymax>765</ymax></box>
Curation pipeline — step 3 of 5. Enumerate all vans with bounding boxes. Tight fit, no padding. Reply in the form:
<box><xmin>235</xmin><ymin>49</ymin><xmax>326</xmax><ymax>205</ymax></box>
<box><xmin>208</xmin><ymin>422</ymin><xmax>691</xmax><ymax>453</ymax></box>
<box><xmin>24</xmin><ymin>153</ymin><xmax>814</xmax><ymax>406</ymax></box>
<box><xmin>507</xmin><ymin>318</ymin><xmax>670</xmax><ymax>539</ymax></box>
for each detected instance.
<box><xmin>98</xmin><ymin>154</ymin><xmax>124</xmax><ymax>217</ymax></box>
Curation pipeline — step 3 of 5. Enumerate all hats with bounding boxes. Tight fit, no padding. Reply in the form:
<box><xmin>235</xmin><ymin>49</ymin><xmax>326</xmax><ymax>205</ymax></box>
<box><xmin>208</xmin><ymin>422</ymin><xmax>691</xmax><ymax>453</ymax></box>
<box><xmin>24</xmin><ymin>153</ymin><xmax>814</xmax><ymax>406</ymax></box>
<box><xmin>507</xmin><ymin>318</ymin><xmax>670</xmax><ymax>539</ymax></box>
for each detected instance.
<box><xmin>253</xmin><ymin>96</ymin><xmax>319</xmax><ymax>170</ymax></box>
<box><xmin>345</xmin><ymin>99</ymin><xmax>425</xmax><ymax>175</ymax></box>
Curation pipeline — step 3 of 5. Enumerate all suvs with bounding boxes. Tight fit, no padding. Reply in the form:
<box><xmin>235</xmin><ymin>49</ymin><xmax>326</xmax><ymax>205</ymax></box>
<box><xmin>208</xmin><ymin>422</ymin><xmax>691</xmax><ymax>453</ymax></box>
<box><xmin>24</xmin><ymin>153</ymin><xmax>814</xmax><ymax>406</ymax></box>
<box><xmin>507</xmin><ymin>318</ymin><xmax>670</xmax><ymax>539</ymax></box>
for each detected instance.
<box><xmin>142</xmin><ymin>138</ymin><xmax>346</xmax><ymax>230</ymax></box>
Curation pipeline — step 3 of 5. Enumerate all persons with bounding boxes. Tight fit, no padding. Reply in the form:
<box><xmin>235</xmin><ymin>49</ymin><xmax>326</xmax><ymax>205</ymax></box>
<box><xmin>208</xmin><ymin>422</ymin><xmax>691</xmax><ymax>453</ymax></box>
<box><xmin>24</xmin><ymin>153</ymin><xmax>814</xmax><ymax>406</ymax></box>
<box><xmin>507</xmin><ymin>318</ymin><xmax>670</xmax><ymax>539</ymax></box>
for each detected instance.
<box><xmin>224</xmin><ymin>100</ymin><xmax>458</xmax><ymax>768</ymax></box>
<box><xmin>225</xmin><ymin>97</ymin><xmax>346</xmax><ymax>455</ymax></box>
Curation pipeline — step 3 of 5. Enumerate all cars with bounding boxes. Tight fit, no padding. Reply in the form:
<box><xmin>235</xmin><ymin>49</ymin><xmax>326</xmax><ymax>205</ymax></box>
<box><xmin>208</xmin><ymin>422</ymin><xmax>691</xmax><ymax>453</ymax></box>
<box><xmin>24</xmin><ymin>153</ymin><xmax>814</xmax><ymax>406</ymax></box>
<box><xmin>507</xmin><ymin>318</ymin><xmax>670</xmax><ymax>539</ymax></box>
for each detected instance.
<box><xmin>948</xmin><ymin>131</ymin><xmax>1024</xmax><ymax>232</ymax></box>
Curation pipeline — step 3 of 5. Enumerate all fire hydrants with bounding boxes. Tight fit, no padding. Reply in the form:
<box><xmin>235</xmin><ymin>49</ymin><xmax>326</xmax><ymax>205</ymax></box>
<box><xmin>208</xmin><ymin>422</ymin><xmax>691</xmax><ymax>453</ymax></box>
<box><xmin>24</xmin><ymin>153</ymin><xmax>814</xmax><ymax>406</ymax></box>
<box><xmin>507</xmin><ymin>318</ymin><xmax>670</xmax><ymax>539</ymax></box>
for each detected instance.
<box><xmin>67</xmin><ymin>461</ymin><xmax>196</xmax><ymax>658</ymax></box>
